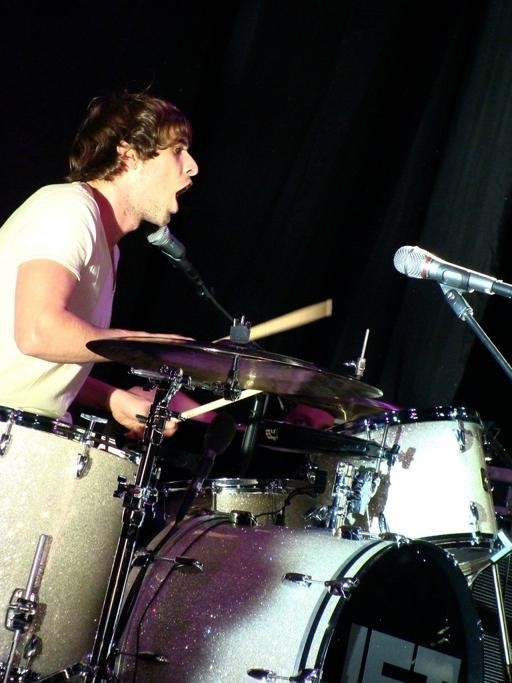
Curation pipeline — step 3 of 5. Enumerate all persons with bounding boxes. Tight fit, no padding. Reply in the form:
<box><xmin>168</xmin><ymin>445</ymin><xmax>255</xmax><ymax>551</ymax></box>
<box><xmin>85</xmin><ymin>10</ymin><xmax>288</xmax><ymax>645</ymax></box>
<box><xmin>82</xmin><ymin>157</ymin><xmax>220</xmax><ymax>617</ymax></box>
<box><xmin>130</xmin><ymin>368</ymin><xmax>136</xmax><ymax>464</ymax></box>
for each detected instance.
<box><xmin>0</xmin><ymin>92</ymin><xmax>199</xmax><ymax>439</ymax></box>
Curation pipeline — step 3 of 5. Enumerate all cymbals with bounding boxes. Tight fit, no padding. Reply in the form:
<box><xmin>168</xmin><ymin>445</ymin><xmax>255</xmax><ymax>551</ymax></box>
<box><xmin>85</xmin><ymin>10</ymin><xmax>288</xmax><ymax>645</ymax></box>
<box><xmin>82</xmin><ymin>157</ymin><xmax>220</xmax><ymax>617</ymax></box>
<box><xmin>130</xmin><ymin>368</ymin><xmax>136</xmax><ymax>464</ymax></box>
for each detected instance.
<box><xmin>249</xmin><ymin>420</ymin><xmax>389</xmax><ymax>458</ymax></box>
<box><xmin>86</xmin><ymin>335</ymin><xmax>385</xmax><ymax>401</ymax></box>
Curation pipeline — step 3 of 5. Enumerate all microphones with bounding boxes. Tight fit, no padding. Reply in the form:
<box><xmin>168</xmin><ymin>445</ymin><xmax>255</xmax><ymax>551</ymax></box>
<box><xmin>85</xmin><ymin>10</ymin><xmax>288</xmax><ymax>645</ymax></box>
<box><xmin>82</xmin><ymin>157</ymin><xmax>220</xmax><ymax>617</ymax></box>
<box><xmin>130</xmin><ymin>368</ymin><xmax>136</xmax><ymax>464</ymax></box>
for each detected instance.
<box><xmin>147</xmin><ymin>225</ymin><xmax>203</xmax><ymax>287</ymax></box>
<box><xmin>392</xmin><ymin>243</ymin><xmax>512</xmax><ymax>299</ymax></box>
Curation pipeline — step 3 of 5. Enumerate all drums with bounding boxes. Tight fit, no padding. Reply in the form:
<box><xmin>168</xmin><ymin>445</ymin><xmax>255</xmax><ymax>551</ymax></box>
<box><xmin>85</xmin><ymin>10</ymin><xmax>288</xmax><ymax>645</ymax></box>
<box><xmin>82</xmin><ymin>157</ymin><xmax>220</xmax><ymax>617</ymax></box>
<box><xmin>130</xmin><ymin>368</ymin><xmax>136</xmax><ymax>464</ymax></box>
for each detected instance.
<box><xmin>1</xmin><ymin>406</ymin><xmax>161</xmax><ymax>683</ymax></box>
<box><xmin>322</xmin><ymin>405</ymin><xmax>500</xmax><ymax>550</ymax></box>
<box><xmin>104</xmin><ymin>512</ymin><xmax>485</xmax><ymax>681</ymax></box>
<box><xmin>208</xmin><ymin>478</ymin><xmax>319</xmax><ymax>529</ymax></box>
